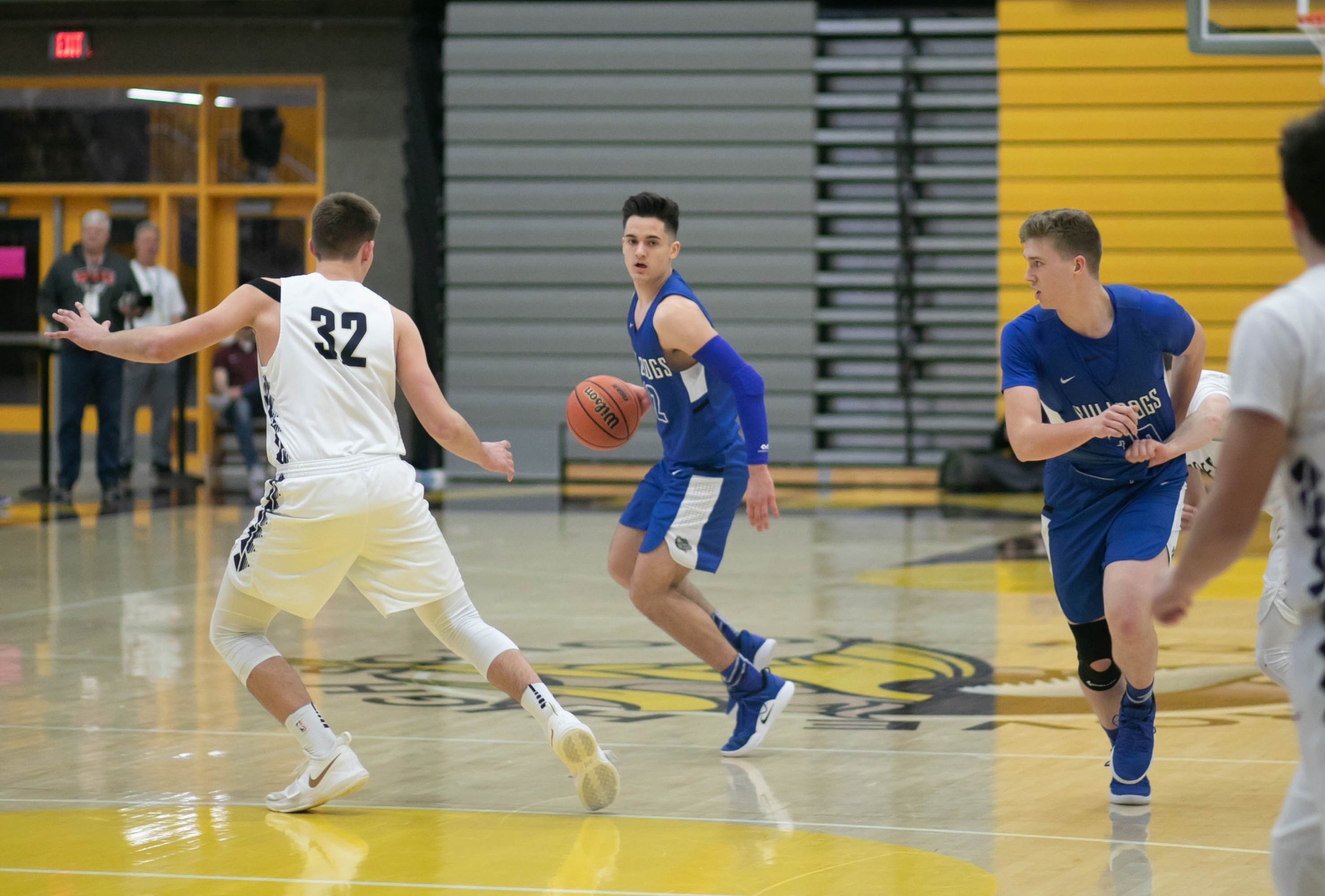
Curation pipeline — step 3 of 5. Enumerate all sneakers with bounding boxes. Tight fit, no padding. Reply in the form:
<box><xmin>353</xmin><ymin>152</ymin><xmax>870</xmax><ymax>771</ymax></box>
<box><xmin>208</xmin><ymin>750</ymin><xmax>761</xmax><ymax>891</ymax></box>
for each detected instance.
<box><xmin>267</xmin><ymin>813</ymin><xmax>368</xmax><ymax>880</ymax></box>
<box><xmin>265</xmin><ymin>731</ymin><xmax>369</xmax><ymax>812</ymax></box>
<box><xmin>737</xmin><ymin>630</ymin><xmax>777</xmax><ymax>671</ymax></box>
<box><xmin>722</xmin><ymin>758</ymin><xmax>794</xmax><ymax>835</ymax></box>
<box><xmin>1104</xmin><ymin>745</ymin><xmax>1150</xmax><ymax>805</ymax></box>
<box><xmin>1110</xmin><ymin>690</ymin><xmax>1157</xmax><ymax>784</ymax></box>
<box><xmin>1108</xmin><ymin>804</ymin><xmax>1152</xmax><ymax>896</ymax></box>
<box><xmin>719</xmin><ymin>667</ymin><xmax>794</xmax><ymax>759</ymax></box>
<box><xmin>546</xmin><ymin>709</ymin><xmax>620</xmax><ymax>811</ymax></box>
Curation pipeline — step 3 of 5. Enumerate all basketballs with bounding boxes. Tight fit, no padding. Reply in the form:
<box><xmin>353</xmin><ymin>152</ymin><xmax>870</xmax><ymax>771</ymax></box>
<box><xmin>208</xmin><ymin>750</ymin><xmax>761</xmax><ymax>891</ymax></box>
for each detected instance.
<box><xmin>566</xmin><ymin>375</ymin><xmax>642</xmax><ymax>451</ymax></box>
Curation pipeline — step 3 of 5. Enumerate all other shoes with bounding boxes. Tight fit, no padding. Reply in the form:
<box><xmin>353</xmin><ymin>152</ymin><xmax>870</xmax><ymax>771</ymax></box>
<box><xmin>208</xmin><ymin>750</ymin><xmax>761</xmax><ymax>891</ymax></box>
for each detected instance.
<box><xmin>48</xmin><ymin>485</ymin><xmax>72</xmax><ymax>502</ymax></box>
<box><xmin>105</xmin><ymin>485</ymin><xmax>123</xmax><ymax>500</ymax></box>
<box><xmin>150</xmin><ymin>463</ymin><xmax>172</xmax><ymax>490</ymax></box>
<box><xmin>205</xmin><ymin>393</ymin><xmax>233</xmax><ymax>418</ymax></box>
<box><xmin>249</xmin><ymin>464</ymin><xmax>270</xmax><ymax>484</ymax></box>
<box><xmin>118</xmin><ymin>465</ymin><xmax>133</xmax><ymax>489</ymax></box>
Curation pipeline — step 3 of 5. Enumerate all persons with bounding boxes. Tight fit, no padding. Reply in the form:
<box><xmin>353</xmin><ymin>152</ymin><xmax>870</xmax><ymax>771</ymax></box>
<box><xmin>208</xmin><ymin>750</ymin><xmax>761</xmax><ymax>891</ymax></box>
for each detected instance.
<box><xmin>116</xmin><ymin>219</ymin><xmax>188</xmax><ymax>499</ymax></box>
<box><xmin>45</xmin><ymin>192</ymin><xmax>620</xmax><ymax>812</ymax></box>
<box><xmin>205</xmin><ymin>326</ymin><xmax>267</xmax><ymax>506</ymax></box>
<box><xmin>606</xmin><ymin>191</ymin><xmax>798</xmax><ymax>757</ymax></box>
<box><xmin>1126</xmin><ymin>369</ymin><xmax>1297</xmax><ymax>701</ymax></box>
<box><xmin>1145</xmin><ymin>105</ymin><xmax>1324</xmax><ymax>896</ymax></box>
<box><xmin>1000</xmin><ymin>207</ymin><xmax>1208</xmax><ymax>805</ymax></box>
<box><xmin>35</xmin><ymin>208</ymin><xmax>146</xmax><ymax>504</ymax></box>
